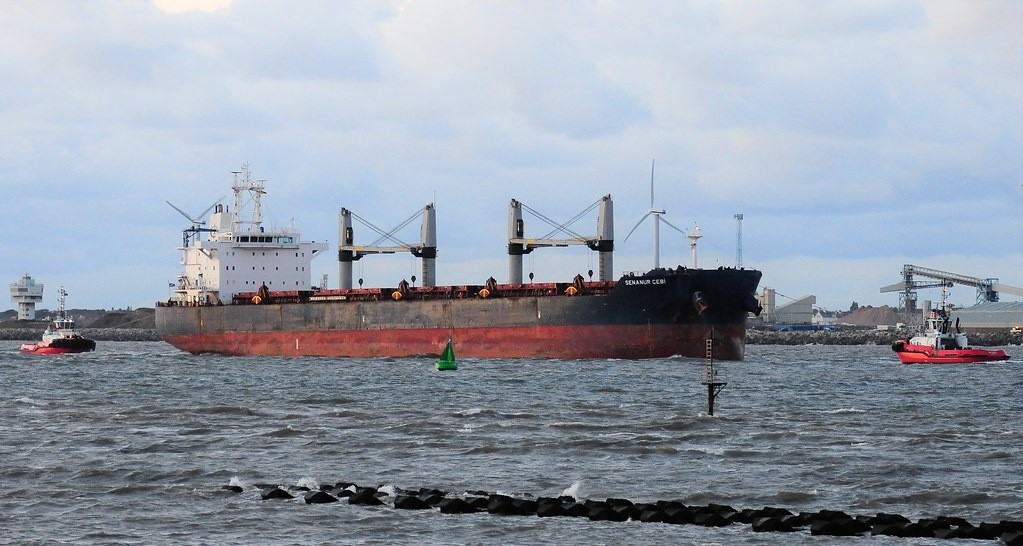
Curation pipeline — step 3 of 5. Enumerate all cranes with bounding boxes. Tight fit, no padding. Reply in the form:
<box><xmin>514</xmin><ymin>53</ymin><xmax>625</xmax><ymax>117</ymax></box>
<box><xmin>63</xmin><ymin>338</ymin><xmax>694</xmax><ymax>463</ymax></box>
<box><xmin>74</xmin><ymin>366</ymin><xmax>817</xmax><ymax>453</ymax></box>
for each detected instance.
<box><xmin>879</xmin><ymin>264</ymin><xmax>999</xmax><ymax>322</ymax></box>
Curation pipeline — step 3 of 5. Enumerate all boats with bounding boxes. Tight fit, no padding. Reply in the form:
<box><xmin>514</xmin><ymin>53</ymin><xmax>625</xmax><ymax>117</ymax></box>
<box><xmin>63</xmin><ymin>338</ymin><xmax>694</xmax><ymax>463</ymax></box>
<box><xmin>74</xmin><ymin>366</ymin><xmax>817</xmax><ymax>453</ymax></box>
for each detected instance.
<box><xmin>435</xmin><ymin>335</ymin><xmax>459</xmax><ymax>371</ymax></box>
<box><xmin>154</xmin><ymin>160</ymin><xmax>762</xmax><ymax>362</ymax></box>
<box><xmin>19</xmin><ymin>315</ymin><xmax>96</xmax><ymax>354</ymax></box>
<box><xmin>888</xmin><ymin>312</ymin><xmax>1010</xmax><ymax>366</ymax></box>
